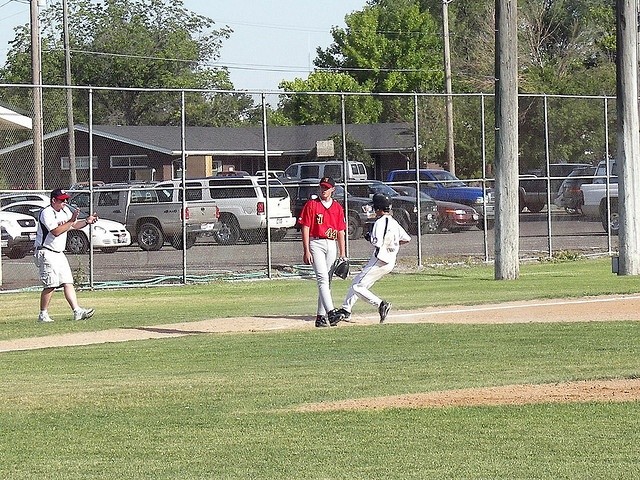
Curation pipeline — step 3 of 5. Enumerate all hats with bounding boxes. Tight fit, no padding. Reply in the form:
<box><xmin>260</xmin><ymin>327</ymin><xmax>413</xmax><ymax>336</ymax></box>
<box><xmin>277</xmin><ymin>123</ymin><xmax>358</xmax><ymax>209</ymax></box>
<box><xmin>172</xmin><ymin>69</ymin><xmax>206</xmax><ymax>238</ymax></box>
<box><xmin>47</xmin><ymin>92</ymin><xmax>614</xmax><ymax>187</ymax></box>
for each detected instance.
<box><xmin>51</xmin><ymin>189</ymin><xmax>71</xmax><ymax>200</ymax></box>
<box><xmin>320</xmin><ymin>177</ymin><xmax>335</xmax><ymax>188</ymax></box>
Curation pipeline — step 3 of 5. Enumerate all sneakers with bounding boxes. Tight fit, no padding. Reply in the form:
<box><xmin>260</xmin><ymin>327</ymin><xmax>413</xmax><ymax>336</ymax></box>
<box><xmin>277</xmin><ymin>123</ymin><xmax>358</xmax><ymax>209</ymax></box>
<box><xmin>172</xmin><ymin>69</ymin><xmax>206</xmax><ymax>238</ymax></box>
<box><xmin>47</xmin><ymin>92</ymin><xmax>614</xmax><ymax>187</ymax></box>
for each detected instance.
<box><xmin>315</xmin><ymin>318</ymin><xmax>329</xmax><ymax>327</ymax></box>
<box><xmin>328</xmin><ymin>309</ymin><xmax>345</xmax><ymax>326</ymax></box>
<box><xmin>39</xmin><ymin>314</ymin><xmax>54</xmax><ymax>322</ymax></box>
<box><xmin>333</xmin><ymin>309</ymin><xmax>350</xmax><ymax>322</ymax></box>
<box><xmin>379</xmin><ymin>300</ymin><xmax>392</xmax><ymax>323</ymax></box>
<box><xmin>74</xmin><ymin>308</ymin><xmax>95</xmax><ymax>321</ymax></box>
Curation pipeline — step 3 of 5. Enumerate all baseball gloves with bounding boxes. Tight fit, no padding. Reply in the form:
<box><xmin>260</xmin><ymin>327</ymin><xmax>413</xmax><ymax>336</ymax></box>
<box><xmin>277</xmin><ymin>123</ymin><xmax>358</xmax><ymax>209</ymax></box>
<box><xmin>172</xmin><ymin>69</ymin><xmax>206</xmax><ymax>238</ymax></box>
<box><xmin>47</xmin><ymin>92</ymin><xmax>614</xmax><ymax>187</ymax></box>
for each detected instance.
<box><xmin>334</xmin><ymin>257</ymin><xmax>349</xmax><ymax>279</ymax></box>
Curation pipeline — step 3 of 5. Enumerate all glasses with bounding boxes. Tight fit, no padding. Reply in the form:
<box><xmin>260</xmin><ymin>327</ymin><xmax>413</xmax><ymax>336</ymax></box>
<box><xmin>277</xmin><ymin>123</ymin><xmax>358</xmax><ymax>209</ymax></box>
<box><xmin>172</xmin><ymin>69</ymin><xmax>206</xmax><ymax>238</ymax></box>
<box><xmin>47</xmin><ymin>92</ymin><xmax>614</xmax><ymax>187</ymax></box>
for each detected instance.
<box><xmin>61</xmin><ymin>198</ymin><xmax>68</xmax><ymax>202</ymax></box>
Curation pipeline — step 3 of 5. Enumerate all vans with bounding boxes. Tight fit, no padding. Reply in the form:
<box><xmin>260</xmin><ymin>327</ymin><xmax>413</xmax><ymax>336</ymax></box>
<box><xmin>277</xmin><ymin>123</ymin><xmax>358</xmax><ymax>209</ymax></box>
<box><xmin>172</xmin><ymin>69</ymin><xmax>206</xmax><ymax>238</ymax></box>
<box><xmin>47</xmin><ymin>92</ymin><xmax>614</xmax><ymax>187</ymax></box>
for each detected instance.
<box><xmin>592</xmin><ymin>159</ymin><xmax>618</xmax><ymax>183</ymax></box>
<box><xmin>277</xmin><ymin>161</ymin><xmax>366</xmax><ymax>183</ymax></box>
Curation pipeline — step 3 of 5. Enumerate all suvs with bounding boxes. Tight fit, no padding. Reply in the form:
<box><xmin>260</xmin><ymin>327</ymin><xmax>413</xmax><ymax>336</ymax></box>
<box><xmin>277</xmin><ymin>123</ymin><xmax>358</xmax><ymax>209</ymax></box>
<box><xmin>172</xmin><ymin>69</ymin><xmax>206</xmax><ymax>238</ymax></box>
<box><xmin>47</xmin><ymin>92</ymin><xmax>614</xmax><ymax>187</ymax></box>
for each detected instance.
<box><xmin>335</xmin><ymin>180</ymin><xmax>438</xmax><ymax>235</ymax></box>
<box><xmin>282</xmin><ymin>179</ymin><xmax>393</xmax><ymax>240</ymax></box>
<box><xmin>154</xmin><ymin>175</ymin><xmax>296</xmax><ymax>246</ymax></box>
<box><xmin>1</xmin><ymin>211</ymin><xmax>38</xmax><ymax>259</ymax></box>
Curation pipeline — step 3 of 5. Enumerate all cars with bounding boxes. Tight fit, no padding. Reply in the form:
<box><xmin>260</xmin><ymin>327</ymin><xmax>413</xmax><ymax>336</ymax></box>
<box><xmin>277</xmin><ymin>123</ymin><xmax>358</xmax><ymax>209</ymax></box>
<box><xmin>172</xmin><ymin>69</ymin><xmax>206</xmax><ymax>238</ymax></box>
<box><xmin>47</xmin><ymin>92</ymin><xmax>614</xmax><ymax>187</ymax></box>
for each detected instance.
<box><xmin>556</xmin><ymin>167</ymin><xmax>597</xmax><ymax>214</ymax></box>
<box><xmin>0</xmin><ymin>192</ymin><xmax>51</xmax><ymax>208</ymax></box>
<box><xmin>388</xmin><ymin>185</ymin><xmax>479</xmax><ymax>234</ymax></box>
<box><xmin>255</xmin><ymin>170</ymin><xmax>285</xmax><ymax>178</ymax></box>
<box><xmin>69</xmin><ymin>181</ymin><xmax>105</xmax><ymax>190</ymax></box>
<box><xmin>216</xmin><ymin>171</ymin><xmax>250</xmax><ymax>176</ymax></box>
<box><xmin>0</xmin><ymin>201</ymin><xmax>131</xmax><ymax>254</ymax></box>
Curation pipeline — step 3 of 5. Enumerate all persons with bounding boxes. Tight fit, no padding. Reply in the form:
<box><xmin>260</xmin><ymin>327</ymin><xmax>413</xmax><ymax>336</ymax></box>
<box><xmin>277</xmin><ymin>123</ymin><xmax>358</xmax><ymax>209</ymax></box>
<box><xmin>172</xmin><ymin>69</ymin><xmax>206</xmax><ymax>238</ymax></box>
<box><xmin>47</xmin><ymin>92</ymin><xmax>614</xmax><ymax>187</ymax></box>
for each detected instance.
<box><xmin>339</xmin><ymin>192</ymin><xmax>412</xmax><ymax>323</ymax></box>
<box><xmin>297</xmin><ymin>176</ymin><xmax>350</xmax><ymax>327</ymax></box>
<box><xmin>33</xmin><ymin>189</ymin><xmax>96</xmax><ymax>323</ymax></box>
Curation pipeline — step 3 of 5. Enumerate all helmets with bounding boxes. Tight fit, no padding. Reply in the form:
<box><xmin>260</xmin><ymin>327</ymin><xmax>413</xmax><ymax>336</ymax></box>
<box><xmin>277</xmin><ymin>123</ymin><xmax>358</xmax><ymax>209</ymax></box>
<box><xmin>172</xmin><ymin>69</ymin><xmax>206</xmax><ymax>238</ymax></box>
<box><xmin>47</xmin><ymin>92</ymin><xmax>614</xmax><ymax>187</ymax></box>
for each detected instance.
<box><xmin>370</xmin><ymin>193</ymin><xmax>392</xmax><ymax>210</ymax></box>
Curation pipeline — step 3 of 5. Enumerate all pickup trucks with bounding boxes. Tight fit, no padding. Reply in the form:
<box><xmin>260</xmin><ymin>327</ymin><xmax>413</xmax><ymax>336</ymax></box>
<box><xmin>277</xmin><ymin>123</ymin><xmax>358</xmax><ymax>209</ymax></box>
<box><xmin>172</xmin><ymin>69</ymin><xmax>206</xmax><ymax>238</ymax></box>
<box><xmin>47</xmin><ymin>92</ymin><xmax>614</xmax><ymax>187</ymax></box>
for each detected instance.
<box><xmin>386</xmin><ymin>169</ymin><xmax>495</xmax><ymax>230</ymax></box>
<box><xmin>579</xmin><ymin>183</ymin><xmax>619</xmax><ymax>236</ymax></box>
<box><xmin>66</xmin><ymin>181</ymin><xmax>222</xmax><ymax>251</ymax></box>
<box><xmin>519</xmin><ymin>164</ymin><xmax>593</xmax><ymax>214</ymax></box>
